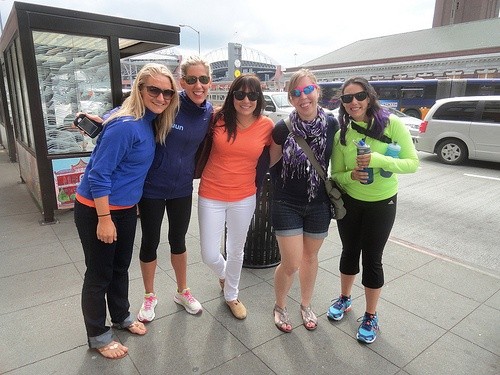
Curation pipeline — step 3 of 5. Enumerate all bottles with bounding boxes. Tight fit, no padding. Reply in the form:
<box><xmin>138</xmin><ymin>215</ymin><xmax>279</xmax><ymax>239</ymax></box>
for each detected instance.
<box><xmin>353</xmin><ymin>137</ymin><xmax>374</xmax><ymax>184</ymax></box>
<box><xmin>379</xmin><ymin>141</ymin><xmax>401</xmax><ymax>178</ymax></box>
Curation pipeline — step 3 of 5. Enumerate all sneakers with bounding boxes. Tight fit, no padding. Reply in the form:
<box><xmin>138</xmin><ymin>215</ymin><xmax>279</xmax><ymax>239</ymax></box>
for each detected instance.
<box><xmin>137</xmin><ymin>290</ymin><xmax>158</xmax><ymax>322</ymax></box>
<box><xmin>356</xmin><ymin>311</ymin><xmax>383</xmax><ymax>343</ymax></box>
<box><xmin>173</xmin><ymin>287</ymin><xmax>203</xmax><ymax>315</ymax></box>
<box><xmin>326</xmin><ymin>294</ymin><xmax>352</xmax><ymax>321</ymax></box>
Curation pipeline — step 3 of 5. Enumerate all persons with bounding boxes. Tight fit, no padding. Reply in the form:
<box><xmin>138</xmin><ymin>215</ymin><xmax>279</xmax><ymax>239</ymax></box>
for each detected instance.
<box><xmin>270</xmin><ymin>68</ymin><xmax>339</xmax><ymax>332</ymax></box>
<box><xmin>71</xmin><ymin>56</ymin><xmax>214</xmax><ymax>322</ymax></box>
<box><xmin>197</xmin><ymin>74</ymin><xmax>275</xmax><ymax>320</ymax></box>
<box><xmin>74</xmin><ymin>63</ymin><xmax>180</xmax><ymax>358</ymax></box>
<box><xmin>326</xmin><ymin>78</ymin><xmax>419</xmax><ymax>343</ymax></box>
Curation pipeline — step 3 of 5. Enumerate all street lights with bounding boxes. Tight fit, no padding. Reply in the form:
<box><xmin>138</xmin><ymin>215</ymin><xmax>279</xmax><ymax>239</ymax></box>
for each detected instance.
<box><xmin>178</xmin><ymin>24</ymin><xmax>201</xmax><ymax>59</ymax></box>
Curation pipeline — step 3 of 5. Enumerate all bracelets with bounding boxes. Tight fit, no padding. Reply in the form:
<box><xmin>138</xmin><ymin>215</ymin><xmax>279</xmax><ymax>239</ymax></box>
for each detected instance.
<box><xmin>97</xmin><ymin>214</ymin><xmax>111</xmax><ymax>217</ymax></box>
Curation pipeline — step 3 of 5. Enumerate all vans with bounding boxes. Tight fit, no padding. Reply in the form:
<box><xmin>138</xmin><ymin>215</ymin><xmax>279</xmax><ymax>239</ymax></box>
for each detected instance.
<box><xmin>414</xmin><ymin>95</ymin><xmax>500</xmax><ymax>165</ymax></box>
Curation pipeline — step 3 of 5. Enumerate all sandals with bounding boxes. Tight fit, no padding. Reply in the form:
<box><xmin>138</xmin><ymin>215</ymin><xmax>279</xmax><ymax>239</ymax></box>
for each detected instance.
<box><xmin>300</xmin><ymin>303</ymin><xmax>318</xmax><ymax>330</ymax></box>
<box><xmin>273</xmin><ymin>304</ymin><xmax>292</xmax><ymax>333</ymax></box>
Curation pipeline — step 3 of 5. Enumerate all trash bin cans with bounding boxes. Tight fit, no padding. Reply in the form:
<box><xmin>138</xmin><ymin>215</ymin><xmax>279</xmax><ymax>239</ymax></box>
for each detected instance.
<box><xmin>223</xmin><ymin>170</ymin><xmax>281</xmax><ymax>269</ymax></box>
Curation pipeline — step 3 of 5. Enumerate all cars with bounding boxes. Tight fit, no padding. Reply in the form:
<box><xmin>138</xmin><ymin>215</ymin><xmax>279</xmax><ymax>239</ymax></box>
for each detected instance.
<box><xmin>330</xmin><ymin>105</ymin><xmax>423</xmax><ymax>143</ymax></box>
<box><xmin>262</xmin><ymin>92</ymin><xmax>334</xmax><ymax>126</ymax></box>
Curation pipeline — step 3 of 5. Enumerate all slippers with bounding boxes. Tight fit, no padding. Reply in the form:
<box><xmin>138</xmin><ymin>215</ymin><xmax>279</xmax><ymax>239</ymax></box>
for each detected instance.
<box><xmin>96</xmin><ymin>339</ymin><xmax>128</xmax><ymax>359</ymax></box>
<box><xmin>126</xmin><ymin>320</ymin><xmax>148</xmax><ymax>336</ymax></box>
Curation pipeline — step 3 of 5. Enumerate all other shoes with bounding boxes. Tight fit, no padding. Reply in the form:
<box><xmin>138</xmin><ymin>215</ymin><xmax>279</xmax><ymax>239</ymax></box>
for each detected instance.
<box><xmin>226</xmin><ymin>298</ymin><xmax>247</xmax><ymax>319</ymax></box>
<box><xmin>219</xmin><ymin>278</ymin><xmax>225</xmax><ymax>289</ymax></box>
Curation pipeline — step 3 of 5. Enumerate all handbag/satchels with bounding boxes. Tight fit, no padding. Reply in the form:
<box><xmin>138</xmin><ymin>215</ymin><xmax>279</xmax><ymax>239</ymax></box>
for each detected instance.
<box><xmin>324</xmin><ymin>180</ymin><xmax>347</xmax><ymax>219</ymax></box>
<box><xmin>193</xmin><ymin>111</ymin><xmax>222</xmax><ymax>179</ymax></box>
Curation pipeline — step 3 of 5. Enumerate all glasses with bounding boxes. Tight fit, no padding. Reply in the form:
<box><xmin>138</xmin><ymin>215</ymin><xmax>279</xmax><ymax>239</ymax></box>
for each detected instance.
<box><xmin>340</xmin><ymin>90</ymin><xmax>368</xmax><ymax>103</ymax></box>
<box><xmin>183</xmin><ymin>75</ymin><xmax>210</xmax><ymax>84</ymax></box>
<box><xmin>290</xmin><ymin>84</ymin><xmax>318</xmax><ymax>98</ymax></box>
<box><xmin>232</xmin><ymin>90</ymin><xmax>260</xmax><ymax>101</ymax></box>
<box><xmin>143</xmin><ymin>84</ymin><xmax>176</xmax><ymax>100</ymax></box>
